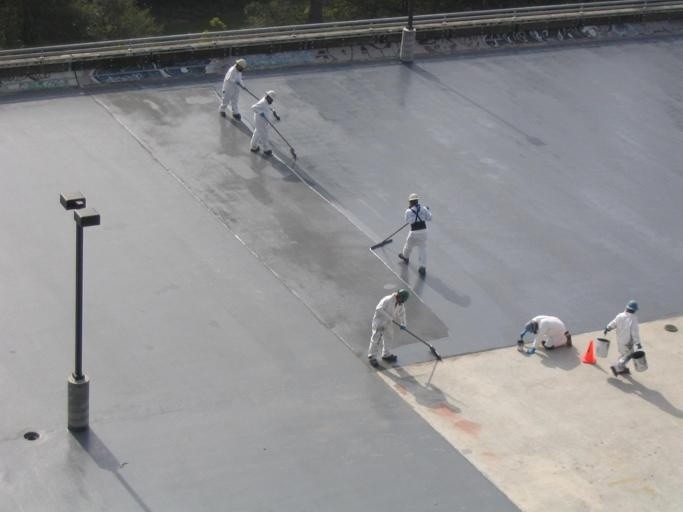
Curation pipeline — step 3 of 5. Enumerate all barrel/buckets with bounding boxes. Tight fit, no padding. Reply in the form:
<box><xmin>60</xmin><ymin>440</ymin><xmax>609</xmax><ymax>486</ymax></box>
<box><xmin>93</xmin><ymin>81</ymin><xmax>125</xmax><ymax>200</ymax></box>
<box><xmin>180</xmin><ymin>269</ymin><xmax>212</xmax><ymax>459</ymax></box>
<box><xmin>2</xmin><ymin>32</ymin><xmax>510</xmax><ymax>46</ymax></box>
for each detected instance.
<box><xmin>594</xmin><ymin>337</ymin><xmax>611</xmax><ymax>358</ymax></box>
<box><xmin>631</xmin><ymin>350</ymin><xmax>648</xmax><ymax>373</ymax></box>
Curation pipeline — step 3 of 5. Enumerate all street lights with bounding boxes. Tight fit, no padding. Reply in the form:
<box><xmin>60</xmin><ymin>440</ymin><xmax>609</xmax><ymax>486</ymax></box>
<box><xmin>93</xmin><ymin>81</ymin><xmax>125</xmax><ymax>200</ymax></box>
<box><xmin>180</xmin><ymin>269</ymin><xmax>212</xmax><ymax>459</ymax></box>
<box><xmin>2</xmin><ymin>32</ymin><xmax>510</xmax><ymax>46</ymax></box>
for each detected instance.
<box><xmin>58</xmin><ymin>189</ymin><xmax>101</xmax><ymax>432</ymax></box>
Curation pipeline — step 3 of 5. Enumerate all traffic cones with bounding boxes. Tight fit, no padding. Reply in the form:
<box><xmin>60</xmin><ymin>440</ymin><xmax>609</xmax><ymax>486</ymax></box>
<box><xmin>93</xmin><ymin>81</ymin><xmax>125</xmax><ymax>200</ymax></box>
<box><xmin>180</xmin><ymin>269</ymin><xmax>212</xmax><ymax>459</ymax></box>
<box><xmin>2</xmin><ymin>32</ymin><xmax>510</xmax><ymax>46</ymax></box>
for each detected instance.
<box><xmin>581</xmin><ymin>340</ymin><xmax>596</xmax><ymax>364</ymax></box>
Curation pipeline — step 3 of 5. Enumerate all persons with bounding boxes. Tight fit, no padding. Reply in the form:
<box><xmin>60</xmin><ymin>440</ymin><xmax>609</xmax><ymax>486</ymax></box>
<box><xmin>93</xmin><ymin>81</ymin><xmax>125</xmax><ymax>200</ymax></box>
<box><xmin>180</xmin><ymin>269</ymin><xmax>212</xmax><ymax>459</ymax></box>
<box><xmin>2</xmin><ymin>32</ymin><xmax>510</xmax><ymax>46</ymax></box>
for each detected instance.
<box><xmin>218</xmin><ymin>58</ymin><xmax>247</xmax><ymax>119</ymax></box>
<box><xmin>603</xmin><ymin>300</ymin><xmax>642</xmax><ymax>377</ymax></box>
<box><xmin>367</xmin><ymin>289</ymin><xmax>409</xmax><ymax>367</ymax></box>
<box><xmin>516</xmin><ymin>315</ymin><xmax>571</xmax><ymax>353</ymax></box>
<box><xmin>398</xmin><ymin>193</ymin><xmax>431</xmax><ymax>278</ymax></box>
<box><xmin>248</xmin><ymin>89</ymin><xmax>279</xmax><ymax>155</ymax></box>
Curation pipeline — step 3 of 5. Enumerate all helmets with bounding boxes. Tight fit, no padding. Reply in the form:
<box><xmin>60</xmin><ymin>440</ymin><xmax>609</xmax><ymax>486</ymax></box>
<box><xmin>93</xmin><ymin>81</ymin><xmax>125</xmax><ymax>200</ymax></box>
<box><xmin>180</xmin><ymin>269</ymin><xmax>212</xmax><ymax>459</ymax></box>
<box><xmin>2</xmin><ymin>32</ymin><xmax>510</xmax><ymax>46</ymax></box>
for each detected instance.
<box><xmin>408</xmin><ymin>193</ymin><xmax>419</xmax><ymax>202</ymax></box>
<box><xmin>524</xmin><ymin>320</ymin><xmax>536</xmax><ymax>332</ymax></box>
<box><xmin>625</xmin><ymin>300</ymin><xmax>639</xmax><ymax>311</ymax></box>
<box><xmin>236</xmin><ymin>59</ymin><xmax>247</xmax><ymax>69</ymax></box>
<box><xmin>266</xmin><ymin>90</ymin><xmax>276</xmax><ymax>101</ymax></box>
<box><xmin>398</xmin><ymin>288</ymin><xmax>409</xmax><ymax>301</ymax></box>
